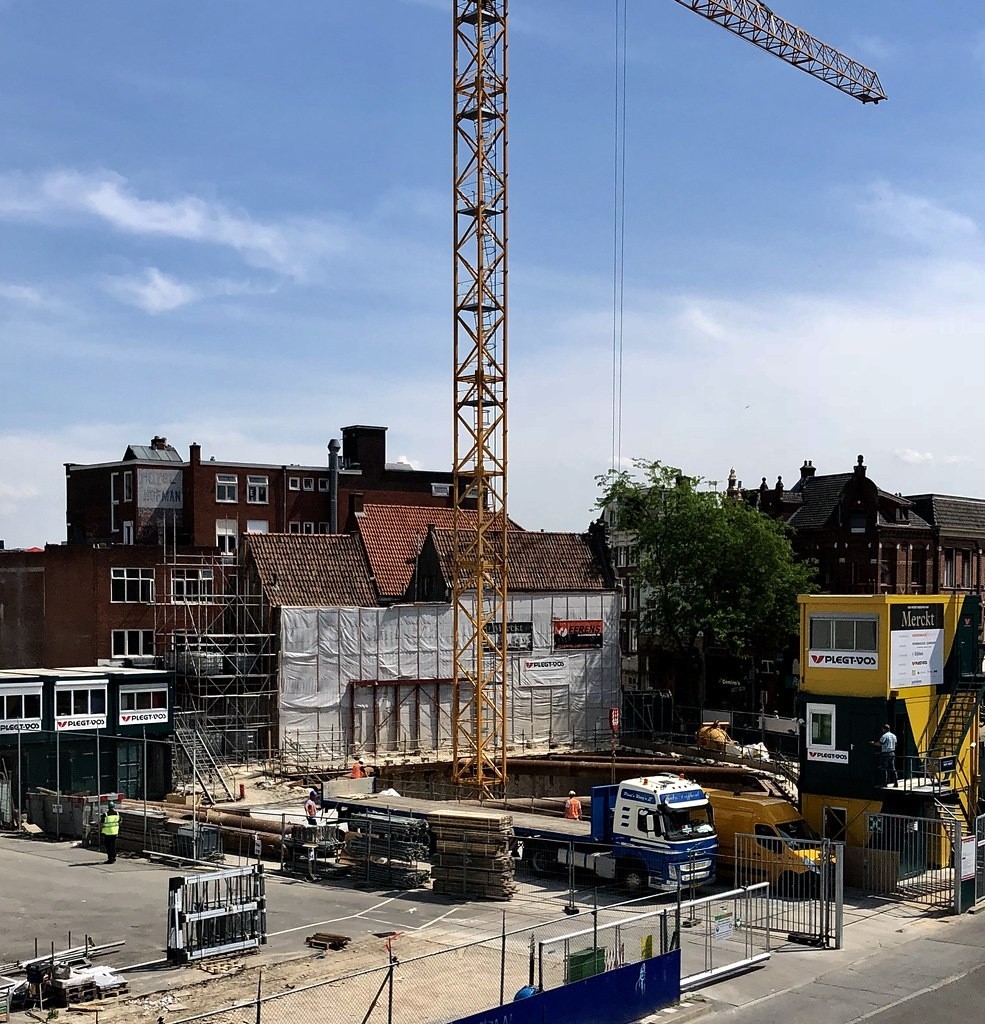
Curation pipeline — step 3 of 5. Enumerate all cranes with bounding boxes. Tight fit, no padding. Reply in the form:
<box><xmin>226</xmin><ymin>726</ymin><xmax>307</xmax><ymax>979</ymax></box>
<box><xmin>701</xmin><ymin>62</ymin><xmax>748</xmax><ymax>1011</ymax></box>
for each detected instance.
<box><xmin>443</xmin><ymin>0</ymin><xmax>887</xmax><ymax>804</ymax></box>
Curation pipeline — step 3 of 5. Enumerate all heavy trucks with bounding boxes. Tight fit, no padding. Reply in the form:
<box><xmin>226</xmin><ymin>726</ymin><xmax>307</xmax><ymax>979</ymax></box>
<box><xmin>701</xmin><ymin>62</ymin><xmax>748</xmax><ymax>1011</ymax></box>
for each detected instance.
<box><xmin>319</xmin><ymin>772</ymin><xmax>724</xmax><ymax>900</ymax></box>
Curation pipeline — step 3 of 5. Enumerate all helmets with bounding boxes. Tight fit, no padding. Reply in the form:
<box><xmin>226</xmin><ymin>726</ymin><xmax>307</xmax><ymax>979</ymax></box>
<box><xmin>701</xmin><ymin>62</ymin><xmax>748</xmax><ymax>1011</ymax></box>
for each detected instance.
<box><xmin>359</xmin><ymin>758</ymin><xmax>364</xmax><ymax>763</ymax></box>
<box><xmin>108</xmin><ymin>801</ymin><xmax>115</xmax><ymax>808</ymax></box>
<box><xmin>569</xmin><ymin>790</ymin><xmax>576</xmax><ymax>795</ymax></box>
<box><xmin>310</xmin><ymin>791</ymin><xmax>317</xmax><ymax>796</ymax></box>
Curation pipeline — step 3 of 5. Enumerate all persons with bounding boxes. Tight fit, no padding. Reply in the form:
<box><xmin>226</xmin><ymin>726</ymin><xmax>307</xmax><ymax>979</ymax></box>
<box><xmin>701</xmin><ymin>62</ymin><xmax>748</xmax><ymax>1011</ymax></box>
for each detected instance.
<box><xmin>101</xmin><ymin>802</ymin><xmax>121</xmax><ymax>864</ymax></box>
<box><xmin>350</xmin><ymin>758</ymin><xmax>368</xmax><ymax>779</ymax></box>
<box><xmin>874</xmin><ymin>724</ymin><xmax>898</xmax><ymax>787</ymax></box>
<box><xmin>304</xmin><ymin>791</ymin><xmax>317</xmax><ymax>825</ymax></box>
<box><xmin>562</xmin><ymin>790</ymin><xmax>582</xmax><ymax>820</ymax></box>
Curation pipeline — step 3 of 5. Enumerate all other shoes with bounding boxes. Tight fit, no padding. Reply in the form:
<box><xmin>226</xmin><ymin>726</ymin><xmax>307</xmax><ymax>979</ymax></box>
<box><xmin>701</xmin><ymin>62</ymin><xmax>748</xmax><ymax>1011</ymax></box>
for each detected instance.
<box><xmin>104</xmin><ymin>859</ymin><xmax>116</xmax><ymax>863</ymax></box>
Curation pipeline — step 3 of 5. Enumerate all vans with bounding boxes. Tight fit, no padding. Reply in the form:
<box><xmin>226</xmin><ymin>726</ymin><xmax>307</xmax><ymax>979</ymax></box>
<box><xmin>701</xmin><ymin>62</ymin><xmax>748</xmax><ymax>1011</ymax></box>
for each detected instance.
<box><xmin>691</xmin><ymin>786</ymin><xmax>840</xmax><ymax>897</ymax></box>
